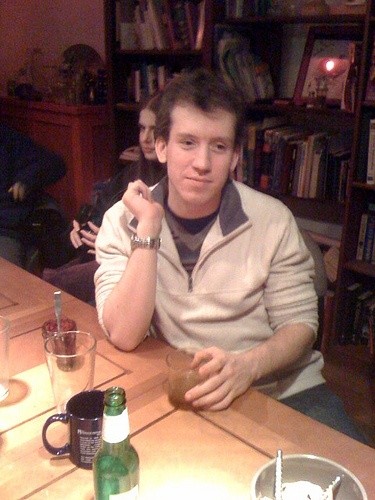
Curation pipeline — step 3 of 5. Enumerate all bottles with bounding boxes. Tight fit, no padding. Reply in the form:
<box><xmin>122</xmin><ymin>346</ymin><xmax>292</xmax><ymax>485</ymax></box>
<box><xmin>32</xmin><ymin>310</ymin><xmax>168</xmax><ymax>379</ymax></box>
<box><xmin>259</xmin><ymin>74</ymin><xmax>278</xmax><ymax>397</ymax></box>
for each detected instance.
<box><xmin>92</xmin><ymin>387</ymin><xmax>141</xmax><ymax>500</ymax></box>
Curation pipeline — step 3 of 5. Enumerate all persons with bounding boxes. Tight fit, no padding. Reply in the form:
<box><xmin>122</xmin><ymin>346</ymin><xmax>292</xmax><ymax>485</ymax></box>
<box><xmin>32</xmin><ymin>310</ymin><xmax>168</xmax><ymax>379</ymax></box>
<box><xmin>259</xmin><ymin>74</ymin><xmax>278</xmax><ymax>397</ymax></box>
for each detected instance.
<box><xmin>41</xmin><ymin>88</ymin><xmax>162</xmax><ymax>304</ymax></box>
<box><xmin>93</xmin><ymin>68</ymin><xmax>369</xmax><ymax>445</ymax></box>
<box><xmin>0</xmin><ymin>130</ymin><xmax>66</xmax><ymax>268</ymax></box>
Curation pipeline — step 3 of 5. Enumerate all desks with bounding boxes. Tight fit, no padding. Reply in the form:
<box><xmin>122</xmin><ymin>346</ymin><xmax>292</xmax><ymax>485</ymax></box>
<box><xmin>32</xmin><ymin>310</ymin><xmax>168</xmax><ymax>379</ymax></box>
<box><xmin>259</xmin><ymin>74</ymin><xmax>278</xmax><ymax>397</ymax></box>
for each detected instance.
<box><xmin>0</xmin><ymin>253</ymin><xmax>375</xmax><ymax>500</ymax></box>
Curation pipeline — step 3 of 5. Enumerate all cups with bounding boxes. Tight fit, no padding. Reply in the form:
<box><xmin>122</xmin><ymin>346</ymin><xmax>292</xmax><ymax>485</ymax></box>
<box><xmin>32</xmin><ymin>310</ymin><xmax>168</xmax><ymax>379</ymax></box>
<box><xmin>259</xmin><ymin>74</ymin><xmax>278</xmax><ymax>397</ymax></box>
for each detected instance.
<box><xmin>251</xmin><ymin>454</ymin><xmax>368</xmax><ymax>500</ymax></box>
<box><xmin>41</xmin><ymin>389</ymin><xmax>105</xmax><ymax>470</ymax></box>
<box><xmin>165</xmin><ymin>348</ymin><xmax>212</xmax><ymax>412</ymax></box>
<box><xmin>40</xmin><ymin>318</ymin><xmax>76</xmax><ymax>371</ymax></box>
<box><xmin>0</xmin><ymin>315</ymin><xmax>11</xmax><ymax>402</ymax></box>
<box><xmin>43</xmin><ymin>330</ymin><xmax>97</xmax><ymax>414</ymax></box>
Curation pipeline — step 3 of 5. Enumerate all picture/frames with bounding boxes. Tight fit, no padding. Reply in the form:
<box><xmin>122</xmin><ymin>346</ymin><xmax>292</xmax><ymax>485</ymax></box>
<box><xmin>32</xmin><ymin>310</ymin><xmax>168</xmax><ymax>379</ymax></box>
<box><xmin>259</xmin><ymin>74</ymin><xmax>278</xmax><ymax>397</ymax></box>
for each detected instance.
<box><xmin>294</xmin><ymin>24</ymin><xmax>363</xmax><ymax>108</ymax></box>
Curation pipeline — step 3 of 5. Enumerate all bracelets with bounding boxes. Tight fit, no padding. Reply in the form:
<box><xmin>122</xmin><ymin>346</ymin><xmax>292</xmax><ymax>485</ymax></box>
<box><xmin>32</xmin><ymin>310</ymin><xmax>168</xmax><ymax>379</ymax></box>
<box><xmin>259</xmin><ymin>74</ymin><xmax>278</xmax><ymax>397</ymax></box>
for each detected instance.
<box><xmin>131</xmin><ymin>235</ymin><xmax>161</xmax><ymax>251</ymax></box>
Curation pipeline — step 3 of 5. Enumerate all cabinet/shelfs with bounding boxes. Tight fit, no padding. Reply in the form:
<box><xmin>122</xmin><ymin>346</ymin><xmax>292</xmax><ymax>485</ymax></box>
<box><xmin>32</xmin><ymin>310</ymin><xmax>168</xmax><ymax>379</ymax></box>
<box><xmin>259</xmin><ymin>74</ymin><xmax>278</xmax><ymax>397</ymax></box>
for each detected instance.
<box><xmin>102</xmin><ymin>0</ymin><xmax>375</xmax><ymax>382</ymax></box>
<box><xmin>0</xmin><ymin>95</ymin><xmax>112</xmax><ymax>220</ymax></box>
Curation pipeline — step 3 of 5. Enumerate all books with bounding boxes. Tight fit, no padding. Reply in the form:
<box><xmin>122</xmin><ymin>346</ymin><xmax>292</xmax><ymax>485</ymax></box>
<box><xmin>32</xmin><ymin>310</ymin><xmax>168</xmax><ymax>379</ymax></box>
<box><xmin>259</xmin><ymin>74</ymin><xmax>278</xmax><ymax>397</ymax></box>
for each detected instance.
<box><xmin>120</xmin><ymin>145</ymin><xmax>141</xmax><ymax>161</ymax></box>
<box><xmin>338</xmin><ymin>37</ymin><xmax>375</xmax><ymax>355</ymax></box>
<box><xmin>125</xmin><ymin>61</ymin><xmax>173</xmax><ymax>104</ymax></box>
<box><xmin>217</xmin><ymin>35</ymin><xmax>275</xmax><ymax>104</ymax></box>
<box><xmin>225</xmin><ymin>0</ymin><xmax>255</xmax><ymax>18</ymax></box>
<box><xmin>119</xmin><ymin>0</ymin><xmax>217</xmax><ymax>52</ymax></box>
<box><xmin>234</xmin><ymin>116</ymin><xmax>352</xmax><ymax>203</ymax></box>
